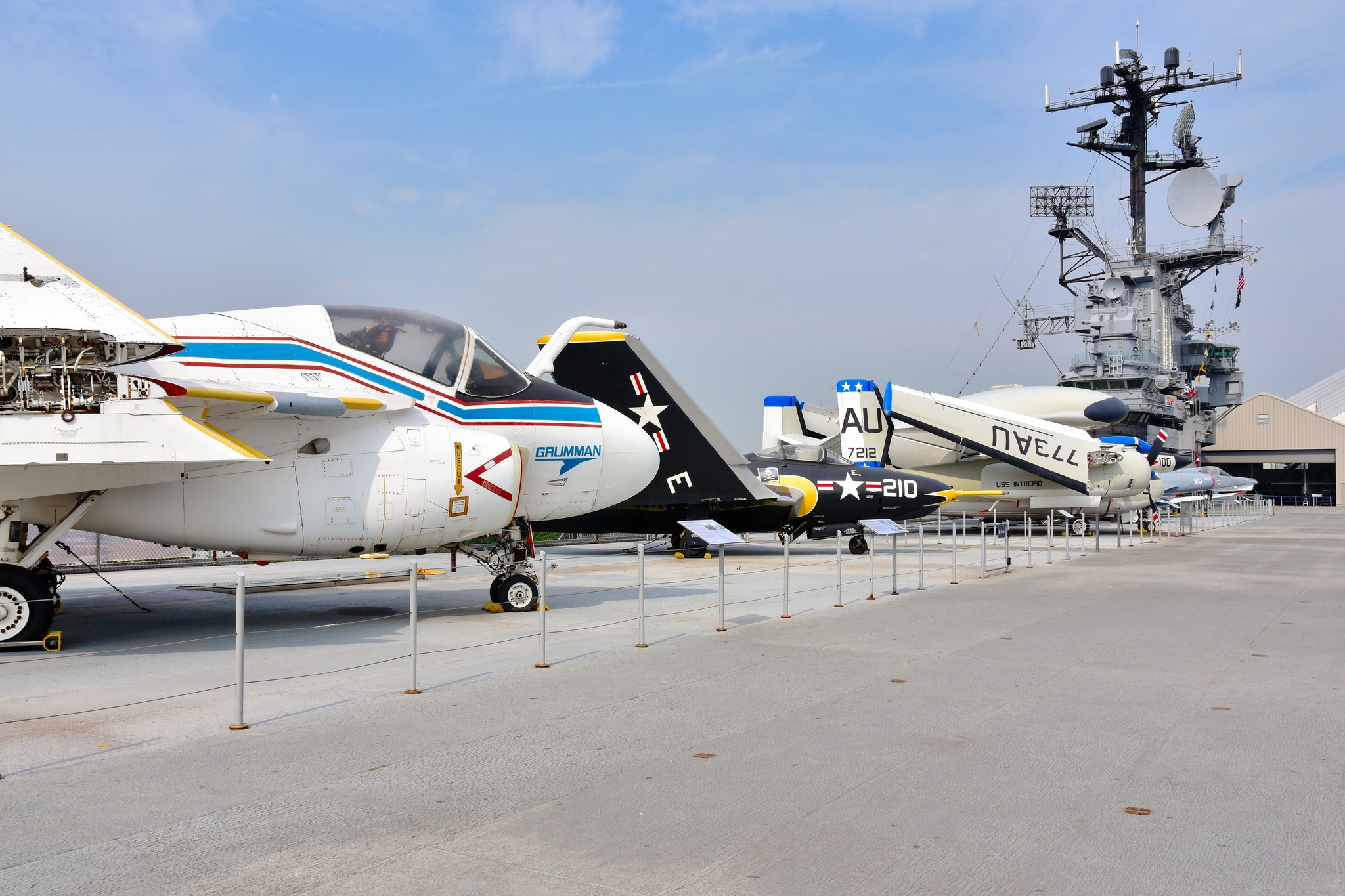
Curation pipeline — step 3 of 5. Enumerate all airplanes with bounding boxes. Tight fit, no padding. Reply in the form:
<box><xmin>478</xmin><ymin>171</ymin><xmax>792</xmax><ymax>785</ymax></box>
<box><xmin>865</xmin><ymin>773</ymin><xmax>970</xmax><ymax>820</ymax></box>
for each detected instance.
<box><xmin>2</xmin><ymin>222</ymin><xmax>661</xmax><ymax>659</ymax></box>
<box><xmin>534</xmin><ymin>328</ymin><xmax>1014</xmax><ymax>559</ymax></box>
<box><xmin>756</xmin><ymin>383</ymin><xmax>1257</xmax><ymax>532</ymax></box>
